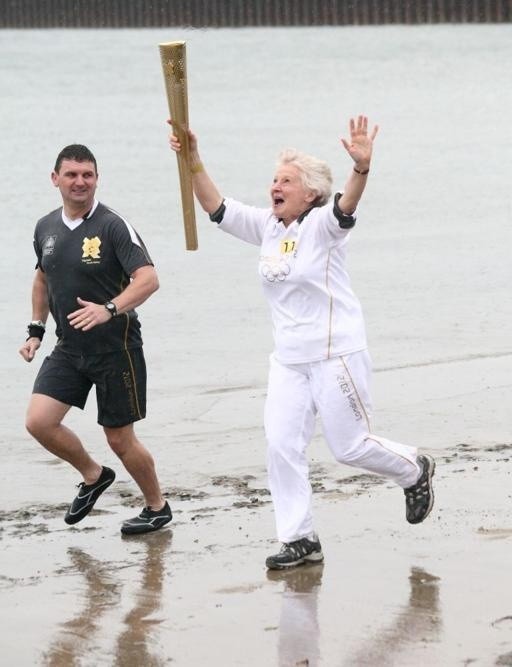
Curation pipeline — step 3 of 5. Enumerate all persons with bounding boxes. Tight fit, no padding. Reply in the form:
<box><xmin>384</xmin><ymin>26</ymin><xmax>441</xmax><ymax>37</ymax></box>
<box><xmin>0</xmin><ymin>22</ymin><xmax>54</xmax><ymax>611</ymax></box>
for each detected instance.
<box><xmin>166</xmin><ymin>113</ymin><xmax>435</xmax><ymax>573</ymax></box>
<box><xmin>17</xmin><ymin>142</ymin><xmax>174</xmax><ymax>535</ymax></box>
<box><xmin>263</xmin><ymin>563</ymin><xmax>444</xmax><ymax>665</ymax></box>
<box><xmin>41</xmin><ymin>528</ymin><xmax>175</xmax><ymax>666</ymax></box>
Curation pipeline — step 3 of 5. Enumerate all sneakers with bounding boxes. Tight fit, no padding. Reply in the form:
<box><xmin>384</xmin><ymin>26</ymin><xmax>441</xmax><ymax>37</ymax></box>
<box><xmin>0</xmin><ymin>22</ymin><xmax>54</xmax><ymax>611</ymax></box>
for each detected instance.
<box><xmin>404</xmin><ymin>454</ymin><xmax>435</xmax><ymax>523</ymax></box>
<box><xmin>65</xmin><ymin>466</ymin><xmax>115</xmax><ymax>524</ymax></box>
<box><xmin>266</xmin><ymin>538</ymin><xmax>324</xmax><ymax>570</ymax></box>
<box><xmin>121</xmin><ymin>501</ymin><xmax>172</xmax><ymax>534</ymax></box>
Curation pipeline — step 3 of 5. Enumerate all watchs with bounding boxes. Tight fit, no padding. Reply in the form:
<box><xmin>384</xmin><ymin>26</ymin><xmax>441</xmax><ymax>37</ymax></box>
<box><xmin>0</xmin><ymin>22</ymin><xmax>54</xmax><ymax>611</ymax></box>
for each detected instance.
<box><xmin>105</xmin><ymin>299</ymin><xmax>117</xmax><ymax>318</ymax></box>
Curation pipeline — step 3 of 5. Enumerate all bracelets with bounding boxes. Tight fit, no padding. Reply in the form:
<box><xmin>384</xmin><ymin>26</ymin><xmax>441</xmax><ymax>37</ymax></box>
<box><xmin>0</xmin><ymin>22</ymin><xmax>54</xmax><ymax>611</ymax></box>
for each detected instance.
<box><xmin>352</xmin><ymin>166</ymin><xmax>370</xmax><ymax>175</ymax></box>
<box><xmin>189</xmin><ymin>160</ymin><xmax>205</xmax><ymax>176</ymax></box>
<box><xmin>25</xmin><ymin>320</ymin><xmax>46</xmax><ymax>341</ymax></box>
